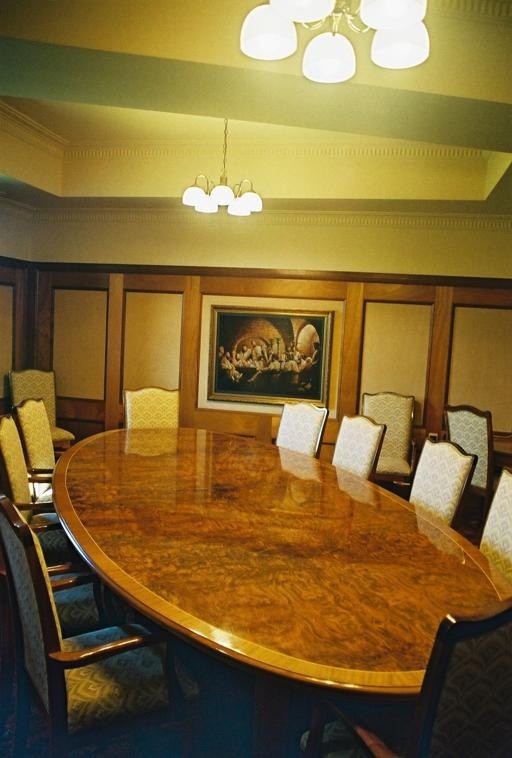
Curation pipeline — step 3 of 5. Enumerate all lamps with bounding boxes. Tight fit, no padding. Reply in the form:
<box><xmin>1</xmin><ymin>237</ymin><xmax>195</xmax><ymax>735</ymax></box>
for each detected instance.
<box><xmin>238</xmin><ymin>1</ymin><xmax>433</xmax><ymax>86</ymax></box>
<box><xmin>180</xmin><ymin>117</ymin><xmax>265</xmax><ymax>216</ymax></box>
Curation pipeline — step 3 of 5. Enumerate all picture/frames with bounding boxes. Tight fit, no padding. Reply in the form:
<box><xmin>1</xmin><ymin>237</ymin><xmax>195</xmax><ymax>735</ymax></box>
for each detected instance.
<box><xmin>206</xmin><ymin>304</ymin><xmax>335</xmax><ymax>408</ymax></box>
<box><xmin>203</xmin><ymin>429</ymin><xmax>326</xmax><ymax>517</ymax></box>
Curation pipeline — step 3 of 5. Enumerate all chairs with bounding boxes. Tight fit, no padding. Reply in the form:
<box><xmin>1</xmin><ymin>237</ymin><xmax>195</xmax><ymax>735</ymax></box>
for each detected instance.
<box><xmin>8</xmin><ymin>368</ymin><xmax>75</xmax><ymax>458</ymax></box>
<box><xmin>409</xmin><ymin>438</ymin><xmax>478</xmax><ymax>528</ymax></box>
<box><xmin>123</xmin><ymin>386</ymin><xmax>180</xmax><ymax>428</ymax></box>
<box><xmin>11</xmin><ymin>396</ymin><xmax>68</xmax><ymax>511</ymax></box>
<box><xmin>362</xmin><ymin>391</ymin><xmax>416</xmax><ymax>489</ymax></box>
<box><xmin>280</xmin><ymin>447</ymin><xmax>321</xmax><ymax>484</ymax></box>
<box><xmin>331</xmin><ymin>415</ymin><xmax>387</xmax><ymax>481</ymax></box>
<box><xmin>0</xmin><ymin>413</ymin><xmax>71</xmax><ymax>551</ymax></box>
<box><xmin>443</xmin><ymin>404</ymin><xmax>502</xmax><ymax>525</ymax></box>
<box><xmin>478</xmin><ymin>466</ymin><xmax>512</xmax><ymax>585</ymax></box>
<box><xmin>125</xmin><ymin>430</ymin><xmax>178</xmax><ymax>456</ymax></box>
<box><xmin>299</xmin><ymin>603</ymin><xmax>512</xmax><ymax>758</ymax></box>
<box><xmin>275</xmin><ymin>401</ymin><xmax>328</xmax><ymax>457</ymax></box>
<box><xmin>1</xmin><ymin>521</ymin><xmax>92</xmax><ymax>670</ymax></box>
<box><xmin>414</xmin><ymin>505</ymin><xmax>467</xmax><ymax>565</ymax></box>
<box><xmin>334</xmin><ymin>466</ymin><xmax>380</xmax><ymax>510</ymax></box>
<box><xmin>0</xmin><ymin>495</ymin><xmax>203</xmax><ymax>757</ymax></box>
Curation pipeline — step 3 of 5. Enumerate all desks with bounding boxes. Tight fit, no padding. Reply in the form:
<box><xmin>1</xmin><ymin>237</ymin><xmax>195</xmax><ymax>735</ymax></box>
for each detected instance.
<box><xmin>51</xmin><ymin>426</ymin><xmax>510</xmax><ymax>758</ymax></box>
